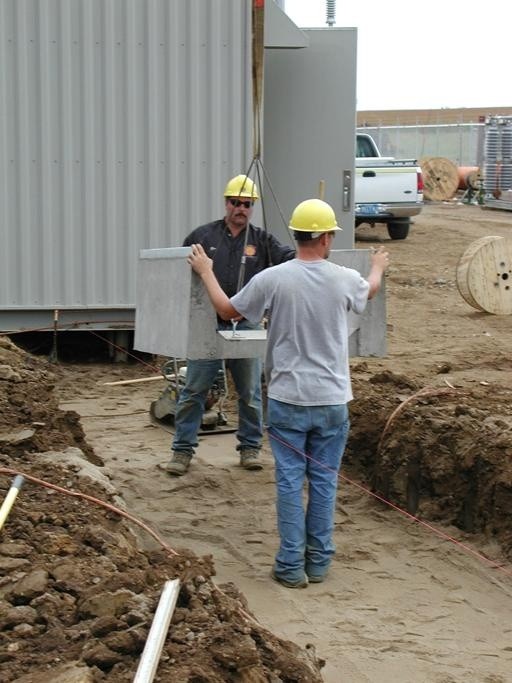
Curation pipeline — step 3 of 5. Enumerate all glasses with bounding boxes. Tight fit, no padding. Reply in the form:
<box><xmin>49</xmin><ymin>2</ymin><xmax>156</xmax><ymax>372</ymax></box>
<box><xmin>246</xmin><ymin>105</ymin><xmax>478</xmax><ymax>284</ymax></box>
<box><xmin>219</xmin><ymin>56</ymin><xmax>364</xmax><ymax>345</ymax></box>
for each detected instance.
<box><xmin>229</xmin><ymin>199</ymin><xmax>254</xmax><ymax>208</ymax></box>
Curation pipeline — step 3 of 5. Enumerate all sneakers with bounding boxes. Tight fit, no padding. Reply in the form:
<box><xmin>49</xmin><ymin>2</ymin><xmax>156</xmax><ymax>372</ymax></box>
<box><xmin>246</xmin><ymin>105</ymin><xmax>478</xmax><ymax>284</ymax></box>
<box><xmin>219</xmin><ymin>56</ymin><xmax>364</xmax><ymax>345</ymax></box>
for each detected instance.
<box><xmin>240</xmin><ymin>448</ymin><xmax>264</xmax><ymax>470</ymax></box>
<box><xmin>166</xmin><ymin>450</ymin><xmax>193</xmax><ymax>476</ymax></box>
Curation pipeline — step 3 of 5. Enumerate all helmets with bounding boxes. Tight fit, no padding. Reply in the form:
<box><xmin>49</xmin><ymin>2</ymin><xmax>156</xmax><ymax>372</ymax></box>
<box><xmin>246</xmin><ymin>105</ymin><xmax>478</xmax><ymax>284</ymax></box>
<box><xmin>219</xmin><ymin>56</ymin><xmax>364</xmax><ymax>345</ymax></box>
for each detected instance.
<box><xmin>224</xmin><ymin>175</ymin><xmax>259</xmax><ymax>198</ymax></box>
<box><xmin>288</xmin><ymin>199</ymin><xmax>343</xmax><ymax>233</ymax></box>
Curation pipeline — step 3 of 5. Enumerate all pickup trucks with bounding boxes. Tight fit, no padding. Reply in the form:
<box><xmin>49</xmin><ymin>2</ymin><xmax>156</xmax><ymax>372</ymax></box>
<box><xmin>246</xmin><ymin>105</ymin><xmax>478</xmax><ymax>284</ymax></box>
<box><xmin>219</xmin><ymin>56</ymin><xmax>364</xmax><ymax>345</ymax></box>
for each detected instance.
<box><xmin>355</xmin><ymin>133</ymin><xmax>424</xmax><ymax>240</ymax></box>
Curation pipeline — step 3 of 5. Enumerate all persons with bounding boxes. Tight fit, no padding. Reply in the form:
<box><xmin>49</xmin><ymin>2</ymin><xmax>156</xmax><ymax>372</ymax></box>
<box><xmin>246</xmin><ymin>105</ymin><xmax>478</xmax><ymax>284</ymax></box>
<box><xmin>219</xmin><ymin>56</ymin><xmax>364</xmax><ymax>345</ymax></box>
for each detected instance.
<box><xmin>185</xmin><ymin>198</ymin><xmax>390</xmax><ymax>589</ymax></box>
<box><xmin>166</xmin><ymin>176</ymin><xmax>297</xmax><ymax>475</ymax></box>
